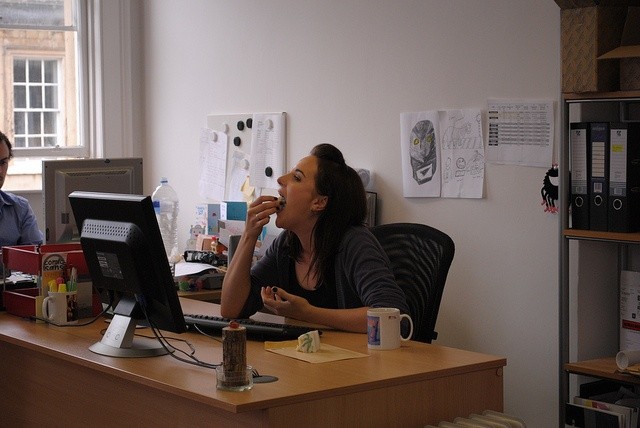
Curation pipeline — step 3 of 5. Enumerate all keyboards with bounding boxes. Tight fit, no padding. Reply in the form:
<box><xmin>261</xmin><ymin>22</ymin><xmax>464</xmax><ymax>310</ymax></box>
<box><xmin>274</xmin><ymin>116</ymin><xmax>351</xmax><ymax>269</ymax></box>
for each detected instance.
<box><xmin>185</xmin><ymin>314</ymin><xmax>323</xmax><ymax>339</ymax></box>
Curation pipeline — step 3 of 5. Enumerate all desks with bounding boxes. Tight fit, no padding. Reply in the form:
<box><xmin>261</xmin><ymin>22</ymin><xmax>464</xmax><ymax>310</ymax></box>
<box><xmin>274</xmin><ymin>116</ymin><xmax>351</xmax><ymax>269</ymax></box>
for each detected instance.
<box><xmin>1</xmin><ymin>297</ymin><xmax>508</xmax><ymax>427</ymax></box>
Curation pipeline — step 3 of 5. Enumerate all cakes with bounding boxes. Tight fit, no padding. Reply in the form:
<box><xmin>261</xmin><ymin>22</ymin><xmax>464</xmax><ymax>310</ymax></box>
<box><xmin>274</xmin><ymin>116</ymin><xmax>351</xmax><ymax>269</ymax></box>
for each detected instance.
<box><xmin>276</xmin><ymin>196</ymin><xmax>286</xmax><ymax>206</ymax></box>
<box><xmin>297</xmin><ymin>330</ymin><xmax>322</xmax><ymax>353</ymax></box>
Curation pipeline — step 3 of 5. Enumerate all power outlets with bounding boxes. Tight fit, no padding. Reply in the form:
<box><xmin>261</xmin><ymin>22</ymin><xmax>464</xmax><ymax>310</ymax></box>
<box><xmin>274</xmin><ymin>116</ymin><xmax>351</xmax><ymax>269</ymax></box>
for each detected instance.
<box><xmin>194</xmin><ymin>204</ymin><xmax>208</xmax><ymax>218</ymax></box>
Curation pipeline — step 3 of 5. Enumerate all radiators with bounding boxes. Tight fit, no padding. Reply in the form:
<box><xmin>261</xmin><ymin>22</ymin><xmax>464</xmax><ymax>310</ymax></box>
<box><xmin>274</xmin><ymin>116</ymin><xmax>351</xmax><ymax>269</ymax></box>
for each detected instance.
<box><xmin>431</xmin><ymin>410</ymin><xmax>524</xmax><ymax>428</ymax></box>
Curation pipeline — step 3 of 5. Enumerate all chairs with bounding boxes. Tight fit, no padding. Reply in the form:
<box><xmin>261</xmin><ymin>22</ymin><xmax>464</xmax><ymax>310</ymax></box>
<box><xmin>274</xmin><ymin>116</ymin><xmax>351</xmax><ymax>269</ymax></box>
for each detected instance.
<box><xmin>372</xmin><ymin>223</ymin><xmax>455</xmax><ymax>345</ymax></box>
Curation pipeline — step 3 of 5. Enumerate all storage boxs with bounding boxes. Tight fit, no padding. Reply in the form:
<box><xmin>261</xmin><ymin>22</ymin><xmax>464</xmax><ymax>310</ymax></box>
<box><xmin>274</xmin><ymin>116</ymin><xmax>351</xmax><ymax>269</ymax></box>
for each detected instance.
<box><xmin>559</xmin><ymin>3</ymin><xmax>638</xmax><ymax>96</ymax></box>
<box><xmin>3</xmin><ymin>243</ymin><xmax>104</xmax><ymax>326</ymax></box>
<box><xmin>556</xmin><ymin>0</ymin><xmax>640</xmax><ymax>95</ymax></box>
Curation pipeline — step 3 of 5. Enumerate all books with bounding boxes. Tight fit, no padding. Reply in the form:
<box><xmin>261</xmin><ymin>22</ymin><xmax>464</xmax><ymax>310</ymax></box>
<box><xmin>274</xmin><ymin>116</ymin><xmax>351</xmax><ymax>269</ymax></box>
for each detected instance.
<box><xmin>564</xmin><ymin>388</ymin><xmax>639</xmax><ymax>428</ymax></box>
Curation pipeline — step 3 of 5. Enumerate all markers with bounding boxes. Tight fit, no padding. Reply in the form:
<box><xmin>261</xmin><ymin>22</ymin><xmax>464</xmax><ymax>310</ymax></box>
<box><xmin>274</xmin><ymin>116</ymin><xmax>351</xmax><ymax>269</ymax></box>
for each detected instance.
<box><xmin>48</xmin><ymin>278</ymin><xmax>67</xmax><ymax>293</ymax></box>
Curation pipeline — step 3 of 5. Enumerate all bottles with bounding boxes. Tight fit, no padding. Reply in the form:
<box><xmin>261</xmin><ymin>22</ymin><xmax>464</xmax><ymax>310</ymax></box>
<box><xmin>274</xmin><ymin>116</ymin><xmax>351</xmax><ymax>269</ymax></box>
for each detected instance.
<box><xmin>151</xmin><ymin>177</ymin><xmax>179</xmax><ymax>280</ymax></box>
<box><xmin>367</xmin><ymin>308</ymin><xmax>414</xmax><ymax>350</ymax></box>
<box><xmin>152</xmin><ymin>200</ymin><xmax>168</xmax><ymax>254</ymax></box>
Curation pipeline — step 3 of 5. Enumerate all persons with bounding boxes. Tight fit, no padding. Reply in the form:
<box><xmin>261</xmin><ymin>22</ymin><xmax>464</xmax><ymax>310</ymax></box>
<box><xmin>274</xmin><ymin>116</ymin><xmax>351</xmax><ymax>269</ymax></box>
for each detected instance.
<box><xmin>0</xmin><ymin>130</ymin><xmax>45</xmax><ymax>247</ymax></box>
<box><xmin>218</xmin><ymin>143</ymin><xmax>408</xmax><ymax>336</ymax></box>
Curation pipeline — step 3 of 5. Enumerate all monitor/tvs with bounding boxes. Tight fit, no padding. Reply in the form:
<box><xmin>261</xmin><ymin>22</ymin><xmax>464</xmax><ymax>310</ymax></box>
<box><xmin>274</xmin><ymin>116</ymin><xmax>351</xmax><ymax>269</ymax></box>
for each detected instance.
<box><xmin>43</xmin><ymin>158</ymin><xmax>144</xmax><ymax>245</ymax></box>
<box><xmin>69</xmin><ymin>190</ymin><xmax>186</xmax><ymax>358</ymax></box>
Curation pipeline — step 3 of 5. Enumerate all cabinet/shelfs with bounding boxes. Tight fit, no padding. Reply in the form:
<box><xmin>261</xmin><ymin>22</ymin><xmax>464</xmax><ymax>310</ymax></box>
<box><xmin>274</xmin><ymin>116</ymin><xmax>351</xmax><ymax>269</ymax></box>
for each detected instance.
<box><xmin>559</xmin><ymin>95</ymin><xmax>640</xmax><ymax>425</ymax></box>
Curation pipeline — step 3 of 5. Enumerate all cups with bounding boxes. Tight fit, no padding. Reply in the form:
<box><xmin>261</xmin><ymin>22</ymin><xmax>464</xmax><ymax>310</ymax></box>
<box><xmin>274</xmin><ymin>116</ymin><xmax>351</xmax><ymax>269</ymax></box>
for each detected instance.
<box><xmin>42</xmin><ymin>291</ymin><xmax>79</xmax><ymax>326</ymax></box>
